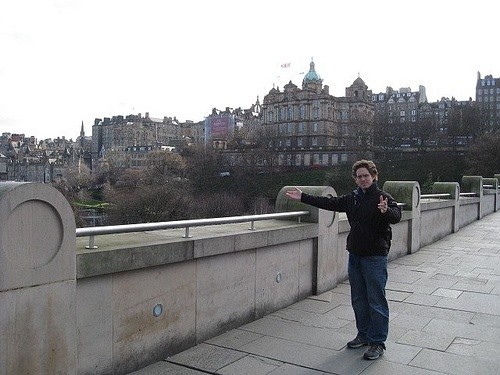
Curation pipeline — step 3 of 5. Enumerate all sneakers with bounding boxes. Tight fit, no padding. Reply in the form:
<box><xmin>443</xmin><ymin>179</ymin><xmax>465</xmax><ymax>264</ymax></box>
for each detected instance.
<box><xmin>364</xmin><ymin>344</ymin><xmax>384</xmax><ymax>360</ymax></box>
<box><xmin>347</xmin><ymin>336</ymin><xmax>368</xmax><ymax>349</ymax></box>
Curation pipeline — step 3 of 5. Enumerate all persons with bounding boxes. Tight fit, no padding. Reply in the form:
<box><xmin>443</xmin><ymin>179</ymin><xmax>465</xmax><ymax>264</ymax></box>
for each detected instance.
<box><xmin>286</xmin><ymin>160</ymin><xmax>402</xmax><ymax>361</ymax></box>
